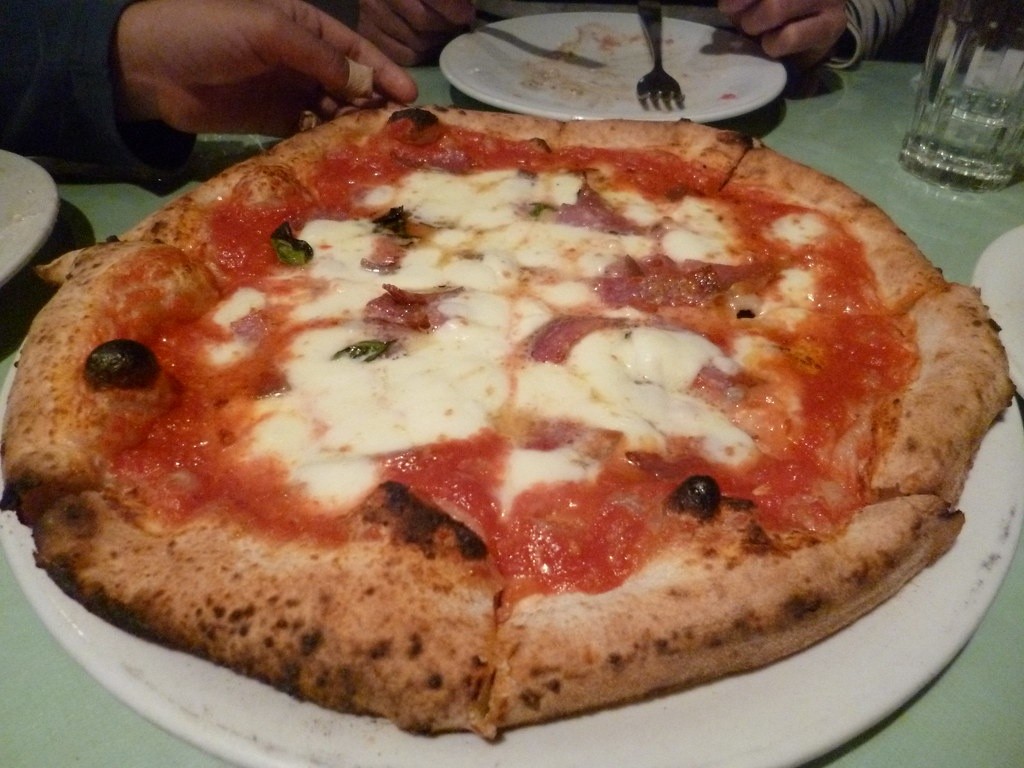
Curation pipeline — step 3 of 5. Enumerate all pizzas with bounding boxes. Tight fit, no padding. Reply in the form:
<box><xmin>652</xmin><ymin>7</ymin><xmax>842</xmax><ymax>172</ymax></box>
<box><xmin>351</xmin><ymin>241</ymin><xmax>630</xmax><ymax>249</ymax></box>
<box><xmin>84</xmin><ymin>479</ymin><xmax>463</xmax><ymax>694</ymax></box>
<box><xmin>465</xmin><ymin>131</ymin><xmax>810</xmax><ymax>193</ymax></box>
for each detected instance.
<box><xmin>6</xmin><ymin>100</ymin><xmax>1017</xmax><ymax>743</ymax></box>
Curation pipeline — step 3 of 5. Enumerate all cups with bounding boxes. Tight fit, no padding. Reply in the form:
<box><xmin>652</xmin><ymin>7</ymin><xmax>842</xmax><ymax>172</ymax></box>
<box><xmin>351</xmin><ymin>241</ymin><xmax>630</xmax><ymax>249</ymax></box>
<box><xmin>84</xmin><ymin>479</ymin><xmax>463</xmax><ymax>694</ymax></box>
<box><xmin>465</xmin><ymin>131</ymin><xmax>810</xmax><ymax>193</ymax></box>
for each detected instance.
<box><xmin>899</xmin><ymin>0</ymin><xmax>1024</xmax><ymax>192</ymax></box>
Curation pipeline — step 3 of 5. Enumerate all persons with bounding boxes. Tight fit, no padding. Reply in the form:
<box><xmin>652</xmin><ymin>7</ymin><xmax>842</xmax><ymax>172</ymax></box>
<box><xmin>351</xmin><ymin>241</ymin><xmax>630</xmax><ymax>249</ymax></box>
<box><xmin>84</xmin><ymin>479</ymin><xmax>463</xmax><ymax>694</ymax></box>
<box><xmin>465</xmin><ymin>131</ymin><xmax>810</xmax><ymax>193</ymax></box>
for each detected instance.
<box><xmin>357</xmin><ymin>0</ymin><xmax>914</xmax><ymax>82</ymax></box>
<box><xmin>0</xmin><ymin>0</ymin><xmax>420</xmax><ymax>190</ymax></box>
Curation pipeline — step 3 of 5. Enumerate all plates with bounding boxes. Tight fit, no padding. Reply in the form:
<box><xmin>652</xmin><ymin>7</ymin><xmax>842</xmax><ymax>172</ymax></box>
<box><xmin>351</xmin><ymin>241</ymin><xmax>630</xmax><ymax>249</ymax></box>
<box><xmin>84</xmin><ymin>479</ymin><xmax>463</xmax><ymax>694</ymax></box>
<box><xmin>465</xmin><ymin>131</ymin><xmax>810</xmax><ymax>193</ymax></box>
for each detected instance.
<box><xmin>0</xmin><ymin>335</ymin><xmax>1024</xmax><ymax>768</ymax></box>
<box><xmin>970</xmin><ymin>225</ymin><xmax>1024</xmax><ymax>399</ymax></box>
<box><xmin>0</xmin><ymin>148</ymin><xmax>60</xmax><ymax>290</ymax></box>
<box><xmin>439</xmin><ymin>12</ymin><xmax>787</xmax><ymax>124</ymax></box>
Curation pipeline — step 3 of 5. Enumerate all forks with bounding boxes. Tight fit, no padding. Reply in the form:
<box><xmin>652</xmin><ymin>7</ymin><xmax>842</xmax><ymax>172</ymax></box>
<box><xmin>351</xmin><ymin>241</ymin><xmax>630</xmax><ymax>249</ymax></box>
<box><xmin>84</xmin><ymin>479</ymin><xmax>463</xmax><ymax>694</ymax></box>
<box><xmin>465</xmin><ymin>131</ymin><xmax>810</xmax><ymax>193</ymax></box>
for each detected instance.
<box><xmin>637</xmin><ymin>0</ymin><xmax>685</xmax><ymax>104</ymax></box>
